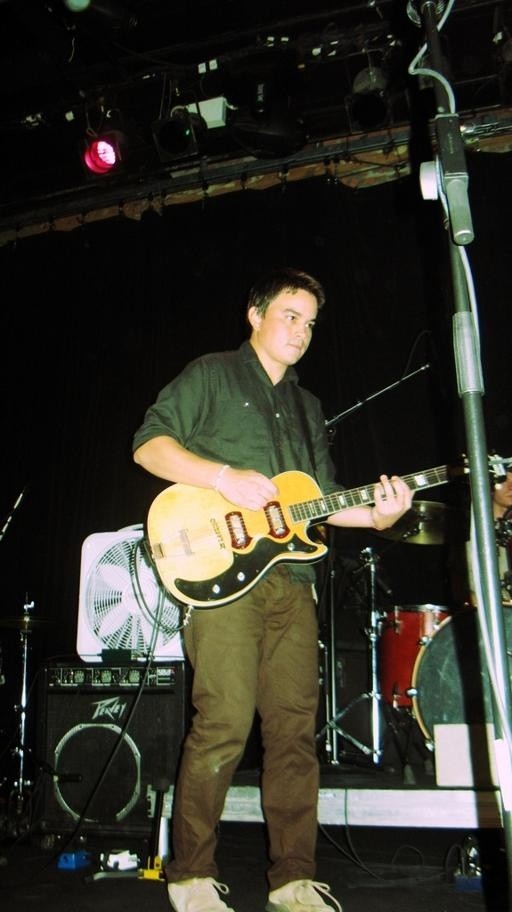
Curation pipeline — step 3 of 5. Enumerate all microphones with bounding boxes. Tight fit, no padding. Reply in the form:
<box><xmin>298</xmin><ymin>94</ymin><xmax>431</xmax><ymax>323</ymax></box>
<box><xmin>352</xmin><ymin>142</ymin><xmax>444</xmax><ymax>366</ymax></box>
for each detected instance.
<box><xmin>58</xmin><ymin>771</ymin><xmax>83</xmax><ymax>784</ymax></box>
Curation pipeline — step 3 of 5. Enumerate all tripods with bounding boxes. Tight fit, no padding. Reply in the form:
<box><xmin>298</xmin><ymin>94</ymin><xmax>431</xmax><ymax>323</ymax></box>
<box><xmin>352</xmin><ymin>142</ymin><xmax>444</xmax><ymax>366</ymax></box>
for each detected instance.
<box><xmin>315</xmin><ymin>549</ymin><xmax>434</xmax><ymax>785</ymax></box>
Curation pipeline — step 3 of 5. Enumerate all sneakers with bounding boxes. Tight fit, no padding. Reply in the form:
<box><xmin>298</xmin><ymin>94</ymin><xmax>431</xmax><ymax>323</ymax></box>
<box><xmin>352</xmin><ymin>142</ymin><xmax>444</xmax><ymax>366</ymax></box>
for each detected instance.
<box><xmin>265</xmin><ymin>879</ymin><xmax>343</xmax><ymax>912</ymax></box>
<box><xmin>167</xmin><ymin>877</ymin><xmax>235</xmax><ymax>912</ymax></box>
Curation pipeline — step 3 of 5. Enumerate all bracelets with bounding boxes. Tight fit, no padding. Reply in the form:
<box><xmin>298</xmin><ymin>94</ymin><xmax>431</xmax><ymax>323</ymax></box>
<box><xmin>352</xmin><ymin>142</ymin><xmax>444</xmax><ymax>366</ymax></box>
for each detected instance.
<box><xmin>212</xmin><ymin>463</ymin><xmax>230</xmax><ymax>491</ymax></box>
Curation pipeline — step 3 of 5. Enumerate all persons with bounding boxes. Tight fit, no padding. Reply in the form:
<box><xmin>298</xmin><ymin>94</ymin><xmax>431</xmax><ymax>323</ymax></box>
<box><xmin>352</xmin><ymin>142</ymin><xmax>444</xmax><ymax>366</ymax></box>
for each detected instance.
<box><xmin>466</xmin><ymin>459</ymin><xmax>512</xmax><ymax>606</ymax></box>
<box><xmin>130</xmin><ymin>269</ymin><xmax>416</xmax><ymax>912</ymax></box>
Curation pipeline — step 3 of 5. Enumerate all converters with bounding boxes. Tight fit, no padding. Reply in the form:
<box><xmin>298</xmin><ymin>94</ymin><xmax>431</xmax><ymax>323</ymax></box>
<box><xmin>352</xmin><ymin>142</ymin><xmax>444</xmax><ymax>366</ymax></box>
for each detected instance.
<box><xmin>100</xmin><ymin>648</ymin><xmax>139</xmax><ymax>662</ymax></box>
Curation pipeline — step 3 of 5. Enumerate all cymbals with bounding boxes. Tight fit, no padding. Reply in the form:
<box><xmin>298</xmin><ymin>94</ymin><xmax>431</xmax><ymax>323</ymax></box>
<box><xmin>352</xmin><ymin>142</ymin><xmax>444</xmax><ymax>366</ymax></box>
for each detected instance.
<box><xmin>382</xmin><ymin>501</ymin><xmax>446</xmax><ymax>546</ymax></box>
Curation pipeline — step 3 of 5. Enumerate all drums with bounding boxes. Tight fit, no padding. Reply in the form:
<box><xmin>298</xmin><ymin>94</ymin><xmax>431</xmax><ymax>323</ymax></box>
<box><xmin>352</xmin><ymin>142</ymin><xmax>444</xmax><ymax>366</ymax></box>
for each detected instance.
<box><xmin>407</xmin><ymin>607</ymin><xmax>511</xmax><ymax>752</ymax></box>
<box><xmin>379</xmin><ymin>604</ymin><xmax>451</xmax><ymax>710</ymax></box>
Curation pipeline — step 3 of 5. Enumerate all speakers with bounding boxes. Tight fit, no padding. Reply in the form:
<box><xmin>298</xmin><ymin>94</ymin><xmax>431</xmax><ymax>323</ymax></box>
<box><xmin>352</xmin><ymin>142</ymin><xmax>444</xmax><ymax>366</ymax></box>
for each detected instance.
<box><xmin>33</xmin><ymin>662</ymin><xmax>197</xmax><ymax>839</ymax></box>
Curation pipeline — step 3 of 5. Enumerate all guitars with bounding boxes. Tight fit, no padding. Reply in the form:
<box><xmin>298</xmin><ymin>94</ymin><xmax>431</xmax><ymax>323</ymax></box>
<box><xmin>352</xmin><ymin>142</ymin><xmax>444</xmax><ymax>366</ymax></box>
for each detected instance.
<box><xmin>146</xmin><ymin>454</ymin><xmax>512</xmax><ymax>609</ymax></box>
